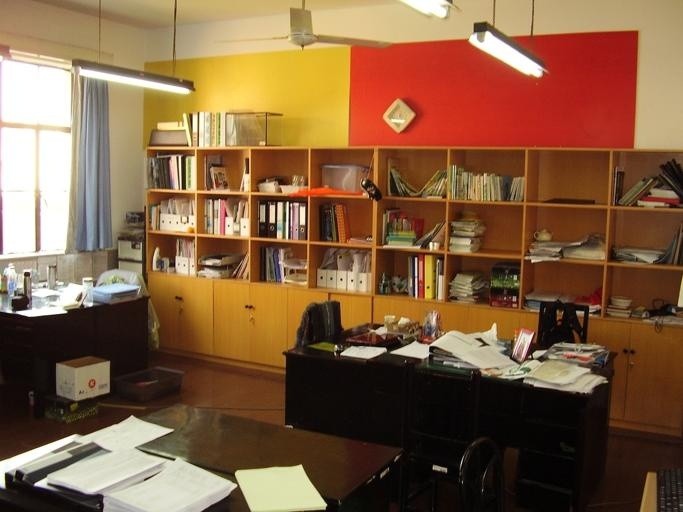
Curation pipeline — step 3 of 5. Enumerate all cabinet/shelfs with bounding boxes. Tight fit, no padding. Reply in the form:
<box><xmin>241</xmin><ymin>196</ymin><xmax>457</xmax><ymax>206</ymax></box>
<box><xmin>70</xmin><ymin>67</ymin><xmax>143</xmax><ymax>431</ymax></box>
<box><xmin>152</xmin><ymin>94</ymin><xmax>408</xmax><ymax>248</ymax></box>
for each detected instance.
<box><xmin>470</xmin><ymin>305</ymin><xmax>561</xmax><ymax>347</ymax></box>
<box><xmin>213</xmin><ymin>279</ymin><xmax>288</xmax><ymax>375</ymax></box>
<box><xmin>288</xmin><ymin>284</ymin><xmax>372</xmax><ymax>352</ymax></box>
<box><xmin>146</xmin><ymin>145</ymin><xmax>681</xmax><ymax>324</ymax></box>
<box><xmin>560</xmin><ymin>312</ymin><xmax>680</xmax><ymax>437</ymax></box>
<box><xmin>144</xmin><ymin>270</ymin><xmax>213</xmax><ymax>363</ymax></box>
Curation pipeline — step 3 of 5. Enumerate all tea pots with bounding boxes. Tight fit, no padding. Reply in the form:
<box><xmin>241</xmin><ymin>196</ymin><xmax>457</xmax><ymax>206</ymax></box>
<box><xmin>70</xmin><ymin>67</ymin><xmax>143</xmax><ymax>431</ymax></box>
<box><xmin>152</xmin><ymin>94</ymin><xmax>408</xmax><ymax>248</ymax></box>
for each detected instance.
<box><xmin>533</xmin><ymin>228</ymin><xmax>554</xmax><ymax>242</ymax></box>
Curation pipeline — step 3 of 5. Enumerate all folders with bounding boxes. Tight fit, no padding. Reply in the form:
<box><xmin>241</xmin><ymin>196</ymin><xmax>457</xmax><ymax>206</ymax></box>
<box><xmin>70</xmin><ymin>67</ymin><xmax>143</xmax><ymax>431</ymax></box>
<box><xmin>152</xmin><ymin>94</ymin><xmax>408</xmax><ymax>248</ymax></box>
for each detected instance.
<box><xmin>256</xmin><ymin>202</ymin><xmax>306</xmax><ymax>241</ymax></box>
<box><xmin>410</xmin><ymin>254</ymin><xmax>441</xmax><ymax>299</ymax></box>
<box><xmin>5</xmin><ymin>441</ymin><xmax>111</xmax><ymax>509</ymax></box>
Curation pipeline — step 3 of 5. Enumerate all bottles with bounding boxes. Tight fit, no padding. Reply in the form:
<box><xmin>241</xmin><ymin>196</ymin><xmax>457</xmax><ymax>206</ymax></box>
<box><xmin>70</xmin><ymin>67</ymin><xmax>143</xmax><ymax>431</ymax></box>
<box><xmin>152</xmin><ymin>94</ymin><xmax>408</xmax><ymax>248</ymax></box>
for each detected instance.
<box><xmin>0</xmin><ymin>263</ymin><xmax>18</xmax><ymax>298</ymax></box>
<box><xmin>82</xmin><ymin>277</ymin><xmax>93</xmax><ymax>307</ymax></box>
<box><xmin>152</xmin><ymin>246</ymin><xmax>161</xmax><ymax>271</ymax></box>
<box><xmin>23</xmin><ymin>272</ymin><xmax>32</xmax><ymax>310</ymax></box>
<box><xmin>45</xmin><ymin>263</ymin><xmax>56</xmax><ymax>289</ymax></box>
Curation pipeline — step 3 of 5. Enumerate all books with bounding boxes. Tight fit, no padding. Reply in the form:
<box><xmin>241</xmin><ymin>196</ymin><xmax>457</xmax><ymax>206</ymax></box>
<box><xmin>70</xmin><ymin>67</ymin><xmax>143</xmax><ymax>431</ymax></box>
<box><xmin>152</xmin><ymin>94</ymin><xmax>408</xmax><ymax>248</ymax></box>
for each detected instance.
<box><xmin>318</xmin><ymin>201</ymin><xmax>352</xmax><ymax>246</ymax></box>
<box><xmin>375</xmin><ymin>156</ymin><xmax>524</xmax><ymax>310</ymax></box>
<box><xmin>252</xmin><ymin>242</ymin><xmax>293</xmax><ymax>283</ymax></box>
<box><xmin>339</xmin><ymin>320</ymin><xmax>611</xmax><ymax>396</ymax></box>
<box><xmin>144</xmin><ymin>112</ymin><xmax>249</xmax><ymax>237</ymax></box>
<box><xmin>610</xmin><ymin>151</ymin><xmax>683</xmax><ymax>267</ymax></box>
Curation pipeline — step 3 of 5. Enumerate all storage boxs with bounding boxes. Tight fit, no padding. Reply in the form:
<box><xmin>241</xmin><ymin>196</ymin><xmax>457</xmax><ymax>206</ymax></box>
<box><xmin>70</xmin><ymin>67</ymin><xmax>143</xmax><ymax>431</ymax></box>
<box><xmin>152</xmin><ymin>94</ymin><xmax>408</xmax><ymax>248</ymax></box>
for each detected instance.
<box><xmin>55</xmin><ymin>356</ymin><xmax>111</xmax><ymax>402</ymax></box>
<box><xmin>111</xmin><ymin>366</ymin><xmax>184</xmax><ymax>403</ymax></box>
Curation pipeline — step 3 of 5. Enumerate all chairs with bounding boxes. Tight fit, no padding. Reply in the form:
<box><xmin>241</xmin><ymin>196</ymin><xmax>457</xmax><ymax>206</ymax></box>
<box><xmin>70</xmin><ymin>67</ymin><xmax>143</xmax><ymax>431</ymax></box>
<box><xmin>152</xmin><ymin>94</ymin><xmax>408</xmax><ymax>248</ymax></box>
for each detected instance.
<box><xmin>459</xmin><ymin>437</ymin><xmax>505</xmax><ymax>512</ymax></box>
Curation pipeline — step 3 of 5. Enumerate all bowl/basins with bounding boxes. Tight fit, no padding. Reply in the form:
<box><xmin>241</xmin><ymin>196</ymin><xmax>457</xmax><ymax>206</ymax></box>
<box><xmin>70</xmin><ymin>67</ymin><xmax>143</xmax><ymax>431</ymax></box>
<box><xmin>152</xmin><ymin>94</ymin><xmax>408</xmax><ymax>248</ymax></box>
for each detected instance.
<box><xmin>609</xmin><ymin>296</ymin><xmax>632</xmax><ymax>307</ymax></box>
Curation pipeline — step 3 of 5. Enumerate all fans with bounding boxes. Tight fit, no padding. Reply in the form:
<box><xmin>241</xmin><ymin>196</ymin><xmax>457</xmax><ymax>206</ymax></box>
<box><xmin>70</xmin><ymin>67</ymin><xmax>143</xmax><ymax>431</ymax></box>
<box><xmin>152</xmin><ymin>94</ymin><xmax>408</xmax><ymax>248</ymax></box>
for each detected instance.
<box><xmin>216</xmin><ymin>0</ymin><xmax>391</xmax><ymax>50</ymax></box>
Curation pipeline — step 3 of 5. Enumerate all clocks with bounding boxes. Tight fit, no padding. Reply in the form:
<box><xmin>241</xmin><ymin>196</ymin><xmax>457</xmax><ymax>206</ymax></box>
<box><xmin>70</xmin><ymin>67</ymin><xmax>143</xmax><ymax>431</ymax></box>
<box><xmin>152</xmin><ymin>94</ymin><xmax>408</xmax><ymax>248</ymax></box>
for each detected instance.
<box><xmin>382</xmin><ymin>98</ymin><xmax>415</xmax><ymax>134</ymax></box>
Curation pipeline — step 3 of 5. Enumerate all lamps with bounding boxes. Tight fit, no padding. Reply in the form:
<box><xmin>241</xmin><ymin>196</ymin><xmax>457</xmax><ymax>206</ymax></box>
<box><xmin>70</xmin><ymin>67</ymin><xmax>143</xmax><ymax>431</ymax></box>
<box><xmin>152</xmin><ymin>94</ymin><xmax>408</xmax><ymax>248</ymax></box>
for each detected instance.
<box><xmin>401</xmin><ymin>0</ymin><xmax>461</xmax><ymax>20</ymax></box>
<box><xmin>72</xmin><ymin>0</ymin><xmax>196</xmax><ymax>94</ymax></box>
<box><xmin>468</xmin><ymin>0</ymin><xmax>550</xmax><ymax>78</ymax></box>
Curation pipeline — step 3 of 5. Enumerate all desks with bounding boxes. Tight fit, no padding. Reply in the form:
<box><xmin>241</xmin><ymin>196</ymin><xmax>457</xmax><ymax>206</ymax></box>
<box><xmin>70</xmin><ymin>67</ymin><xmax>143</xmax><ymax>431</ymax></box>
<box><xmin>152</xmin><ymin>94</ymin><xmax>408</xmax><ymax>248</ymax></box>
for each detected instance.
<box><xmin>0</xmin><ymin>404</ymin><xmax>406</xmax><ymax>512</ymax></box>
<box><xmin>0</xmin><ymin>294</ymin><xmax>151</xmax><ymax>419</ymax></box>
<box><xmin>283</xmin><ymin>323</ymin><xmax>613</xmax><ymax>512</ymax></box>
<box><xmin>373</xmin><ymin>294</ymin><xmax>469</xmax><ymax>336</ymax></box>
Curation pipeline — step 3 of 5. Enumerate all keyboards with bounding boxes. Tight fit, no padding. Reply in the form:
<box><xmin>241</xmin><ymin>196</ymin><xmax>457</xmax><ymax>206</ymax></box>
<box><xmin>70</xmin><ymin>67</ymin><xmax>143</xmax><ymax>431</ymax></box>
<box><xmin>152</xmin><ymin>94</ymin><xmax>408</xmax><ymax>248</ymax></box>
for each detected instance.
<box><xmin>656</xmin><ymin>466</ymin><xmax>680</xmax><ymax>512</ymax></box>
